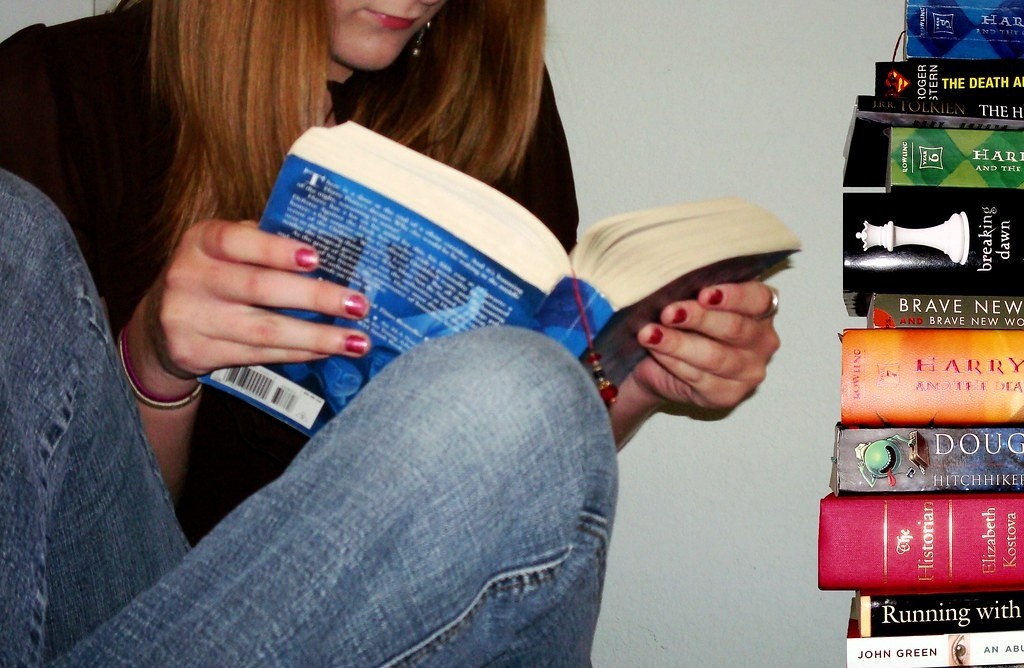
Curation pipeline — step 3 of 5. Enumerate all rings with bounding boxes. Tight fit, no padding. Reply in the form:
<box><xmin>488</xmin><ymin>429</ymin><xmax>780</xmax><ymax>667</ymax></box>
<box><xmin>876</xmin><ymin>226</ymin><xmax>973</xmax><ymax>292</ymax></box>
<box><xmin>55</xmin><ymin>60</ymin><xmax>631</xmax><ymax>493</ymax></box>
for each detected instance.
<box><xmin>756</xmin><ymin>286</ymin><xmax>779</xmax><ymax>319</ymax></box>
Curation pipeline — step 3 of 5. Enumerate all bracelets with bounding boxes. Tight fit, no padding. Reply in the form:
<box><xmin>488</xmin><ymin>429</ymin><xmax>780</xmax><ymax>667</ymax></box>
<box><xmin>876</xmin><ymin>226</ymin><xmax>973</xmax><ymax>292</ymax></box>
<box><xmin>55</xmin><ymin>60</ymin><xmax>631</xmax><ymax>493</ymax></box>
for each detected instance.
<box><xmin>117</xmin><ymin>328</ymin><xmax>204</xmax><ymax>410</ymax></box>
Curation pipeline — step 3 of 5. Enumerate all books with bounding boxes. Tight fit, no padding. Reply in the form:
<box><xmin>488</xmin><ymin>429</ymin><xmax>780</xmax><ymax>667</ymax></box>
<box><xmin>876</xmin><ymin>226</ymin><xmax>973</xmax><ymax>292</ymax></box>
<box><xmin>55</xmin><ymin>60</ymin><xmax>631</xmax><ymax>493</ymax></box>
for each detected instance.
<box><xmin>818</xmin><ymin>0</ymin><xmax>1024</xmax><ymax>668</ymax></box>
<box><xmin>196</xmin><ymin>121</ymin><xmax>803</xmax><ymax>439</ymax></box>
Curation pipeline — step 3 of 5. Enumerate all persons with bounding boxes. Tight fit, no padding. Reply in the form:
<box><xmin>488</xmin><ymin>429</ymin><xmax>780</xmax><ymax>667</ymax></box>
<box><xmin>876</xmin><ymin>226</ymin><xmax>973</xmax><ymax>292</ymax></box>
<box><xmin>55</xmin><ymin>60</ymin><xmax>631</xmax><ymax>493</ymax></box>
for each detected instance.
<box><xmin>1</xmin><ymin>1</ymin><xmax>780</xmax><ymax>667</ymax></box>
